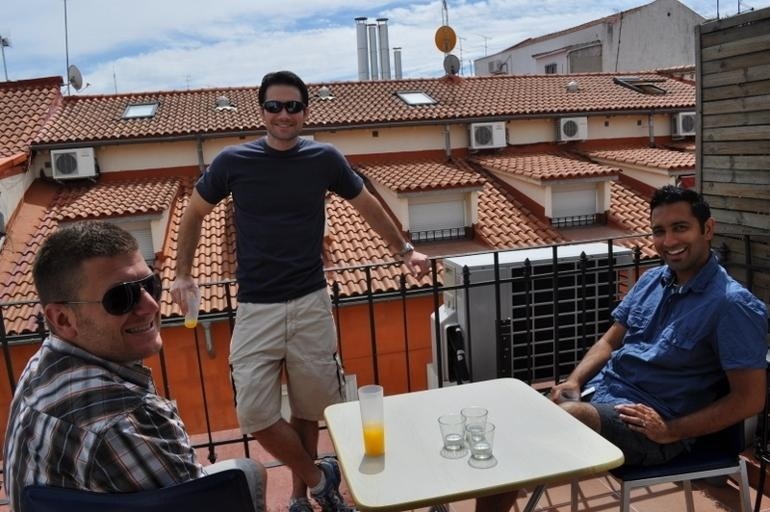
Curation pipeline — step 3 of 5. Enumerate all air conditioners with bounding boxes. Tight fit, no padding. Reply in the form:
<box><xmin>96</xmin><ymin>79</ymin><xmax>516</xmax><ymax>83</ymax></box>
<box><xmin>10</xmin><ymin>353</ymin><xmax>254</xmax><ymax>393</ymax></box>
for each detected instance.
<box><xmin>466</xmin><ymin>121</ymin><xmax>507</xmax><ymax>150</ymax></box>
<box><xmin>672</xmin><ymin>112</ymin><xmax>696</xmax><ymax>137</ymax></box>
<box><xmin>50</xmin><ymin>146</ymin><xmax>99</xmax><ymax>180</ymax></box>
<box><xmin>555</xmin><ymin>115</ymin><xmax>588</xmax><ymax>142</ymax></box>
<box><xmin>426</xmin><ymin>240</ymin><xmax>634</xmax><ymax>389</ymax></box>
<box><xmin>488</xmin><ymin>59</ymin><xmax>501</xmax><ymax>73</ymax></box>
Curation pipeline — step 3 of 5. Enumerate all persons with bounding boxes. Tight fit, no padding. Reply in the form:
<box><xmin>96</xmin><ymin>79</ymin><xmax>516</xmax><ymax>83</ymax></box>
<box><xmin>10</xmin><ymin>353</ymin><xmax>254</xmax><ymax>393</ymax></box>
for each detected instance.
<box><xmin>170</xmin><ymin>71</ymin><xmax>431</xmax><ymax>512</ymax></box>
<box><xmin>476</xmin><ymin>185</ymin><xmax>770</xmax><ymax>512</ymax></box>
<box><xmin>2</xmin><ymin>219</ymin><xmax>267</xmax><ymax>512</ymax></box>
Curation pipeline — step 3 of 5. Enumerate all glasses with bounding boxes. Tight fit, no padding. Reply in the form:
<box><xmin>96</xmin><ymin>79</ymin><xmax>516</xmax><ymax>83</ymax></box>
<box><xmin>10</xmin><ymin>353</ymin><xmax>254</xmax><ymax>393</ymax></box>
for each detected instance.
<box><xmin>58</xmin><ymin>271</ymin><xmax>163</xmax><ymax>318</ymax></box>
<box><xmin>262</xmin><ymin>100</ymin><xmax>307</xmax><ymax>114</ymax></box>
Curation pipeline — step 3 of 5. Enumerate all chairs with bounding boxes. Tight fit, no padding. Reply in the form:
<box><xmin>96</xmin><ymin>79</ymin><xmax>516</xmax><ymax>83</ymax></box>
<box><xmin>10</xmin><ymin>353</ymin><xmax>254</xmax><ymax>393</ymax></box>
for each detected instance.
<box><xmin>18</xmin><ymin>458</ymin><xmax>267</xmax><ymax>512</ymax></box>
<box><xmin>571</xmin><ymin>413</ymin><xmax>759</xmax><ymax>511</ymax></box>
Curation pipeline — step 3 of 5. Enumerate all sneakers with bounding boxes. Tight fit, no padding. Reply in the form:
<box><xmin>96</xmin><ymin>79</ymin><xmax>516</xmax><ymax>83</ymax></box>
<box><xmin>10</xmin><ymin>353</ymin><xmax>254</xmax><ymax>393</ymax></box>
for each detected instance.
<box><xmin>310</xmin><ymin>454</ymin><xmax>352</xmax><ymax>511</ymax></box>
<box><xmin>289</xmin><ymin>497</ymin><xmax>313</xmax><ymax>509</ymax></box>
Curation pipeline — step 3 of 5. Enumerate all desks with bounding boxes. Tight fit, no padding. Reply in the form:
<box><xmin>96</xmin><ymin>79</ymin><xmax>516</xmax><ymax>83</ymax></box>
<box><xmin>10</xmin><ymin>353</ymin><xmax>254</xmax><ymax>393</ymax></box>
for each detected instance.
<box><xmin>324</xmin><ymin>378</ymin><xmax>626</xmax><ymax>511</ymax></box>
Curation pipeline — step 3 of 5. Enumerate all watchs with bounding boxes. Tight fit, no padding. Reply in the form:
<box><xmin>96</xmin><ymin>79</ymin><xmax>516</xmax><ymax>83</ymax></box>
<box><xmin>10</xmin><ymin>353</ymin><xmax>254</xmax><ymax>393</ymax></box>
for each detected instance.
<box><xmin>399</xmin><ymin>241</ymin><xmax>414</xmax><ymax>257</ymax></box>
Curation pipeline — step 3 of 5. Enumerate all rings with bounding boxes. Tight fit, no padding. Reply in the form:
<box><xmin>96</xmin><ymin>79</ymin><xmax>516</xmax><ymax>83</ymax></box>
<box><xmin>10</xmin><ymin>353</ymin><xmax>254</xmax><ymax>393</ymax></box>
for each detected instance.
<box><xmin>642</xmin><ymin>421</ymin><xmax>647</xmax><ymax>427</ymax></box>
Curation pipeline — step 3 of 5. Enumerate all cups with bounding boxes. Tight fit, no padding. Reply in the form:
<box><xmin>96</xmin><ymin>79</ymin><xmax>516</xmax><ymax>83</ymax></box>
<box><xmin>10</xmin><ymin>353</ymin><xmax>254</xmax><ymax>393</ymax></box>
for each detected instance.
<box><xmin>184</xmin><ymin>289</ymin><xmax>202</xmax><ymax>329</ymax></box>
<box><xmin>440</xmin><ymin>446</ymin><xmax>497</xmax><ymax>469</ymax></box>
<box><xmin>358</xmin><ymin>384</ymin><xmax>386</xmax><ymax>458</ymax></box>
<box><xmin>460</xmin><ymin>407</ymin><xmax>488</xmax><ymax>444</ymax></box>
<box><xmin>465</xmin><ymin>422</ymin><xmax>496</xmax><ymax>460</ymax></box>
<box><xmin>559</xmin><ymin>390</ymin><xmax>581</xmax><ymax>403</ymax></box>
<box><xmin>437</xmin><ymin>413</ymin><xmax>466</xmax><ymax>451</ymax></box>
